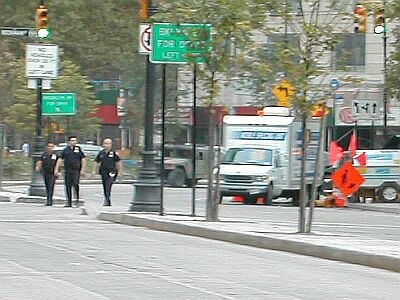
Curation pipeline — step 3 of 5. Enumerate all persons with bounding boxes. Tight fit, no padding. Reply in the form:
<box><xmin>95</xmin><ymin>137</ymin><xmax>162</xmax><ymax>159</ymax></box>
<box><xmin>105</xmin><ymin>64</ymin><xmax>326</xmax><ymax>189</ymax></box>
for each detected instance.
<box><xmin>21</xmin><ymin>141</ymin><xmax>30</xmax><ymax>157</ymax></box>
<box><xmin>54</xmin><ymin>136</ymin><xmax>87</xmax><ymax>207</ymax></box>
<box><xmin>258</xmin><ymin>149</ymin><xmax>275</xmax><ymax>165</ymax></box>
<box><xmin>35</xmin><ymin>142</ymin><xmax>60</xmax><ymax>205</ymax></box>
<box><xmin>92</xmin><ymin>137</ymin><xmax>122</xmax><ymax>206</ymax></box>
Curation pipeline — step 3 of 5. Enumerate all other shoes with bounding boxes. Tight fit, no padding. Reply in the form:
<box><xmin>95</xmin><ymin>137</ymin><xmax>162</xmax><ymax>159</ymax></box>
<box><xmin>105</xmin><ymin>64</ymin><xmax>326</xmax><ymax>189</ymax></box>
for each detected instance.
<box><xmin>104</xmin><ymin>200</ymin><xmax>110</xmax><ymax>206</ymax></box>
<box><xmin>47</xmin><ymin>199</ymin><xmax>52</xmax><ymax>205</ymax></box>
<box><xmin>64</xmin><ymin>204</ymin><xmax>71</xmax><ymax>207</ymax></box>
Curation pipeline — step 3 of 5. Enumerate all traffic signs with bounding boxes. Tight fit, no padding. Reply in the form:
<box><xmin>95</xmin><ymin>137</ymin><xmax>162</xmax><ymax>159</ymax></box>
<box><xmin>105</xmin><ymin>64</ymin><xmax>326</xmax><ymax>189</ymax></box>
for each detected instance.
<box><xmin>41</xmin><ymin>92</ymin><xmax>76</xmax><ymax>116</ymax></box>
<box><xmin>25</xmin><ymin>44</ymin><xmax>59</xmax><ymax>80</ymax></box>
<box><xmin>149</xmin><ymin>23</ymin><xmax>214</xmax><ymax>65</ymax></box>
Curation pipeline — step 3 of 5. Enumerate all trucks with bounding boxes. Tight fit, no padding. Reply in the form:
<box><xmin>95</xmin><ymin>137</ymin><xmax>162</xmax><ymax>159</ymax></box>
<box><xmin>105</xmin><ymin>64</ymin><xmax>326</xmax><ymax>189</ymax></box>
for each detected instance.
<box><xmin>334</xmin><ymin>148</ymin><xmax>400</xmax><ymax>204</ymax></box>
<box><xmin>212</xmin><ymin>107</ymin><xmax>326</xmax><ymax>206</ymax></box>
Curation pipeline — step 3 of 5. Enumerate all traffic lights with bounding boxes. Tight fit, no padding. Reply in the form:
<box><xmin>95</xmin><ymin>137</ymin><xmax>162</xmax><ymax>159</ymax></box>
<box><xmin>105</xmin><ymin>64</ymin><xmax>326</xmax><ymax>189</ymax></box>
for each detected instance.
<box><xmin>373</xmin><ymin>4</ymin><xmax>384</xmax><ymax>35</ymax></box>
<box><xmin>36</xmin><ymin>8</ymin><xmax>49</xmax><ymax>39</ymax></box>
<box><xmin>352</xmin><ymin>4</ymin><xmax>367</xmax><ymax>33</ymax></box>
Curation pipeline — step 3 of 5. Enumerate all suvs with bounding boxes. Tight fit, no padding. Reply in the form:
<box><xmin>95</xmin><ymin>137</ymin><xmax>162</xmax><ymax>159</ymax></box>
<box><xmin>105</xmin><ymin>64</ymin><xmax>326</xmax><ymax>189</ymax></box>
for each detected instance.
<box><xmin>153</xmin><ymin>143</ymin><xmax>211</xmax><ymax>188</ymax></box>
